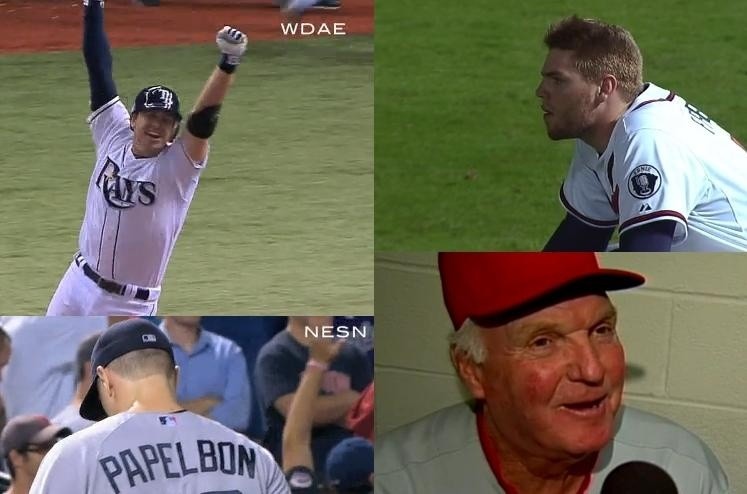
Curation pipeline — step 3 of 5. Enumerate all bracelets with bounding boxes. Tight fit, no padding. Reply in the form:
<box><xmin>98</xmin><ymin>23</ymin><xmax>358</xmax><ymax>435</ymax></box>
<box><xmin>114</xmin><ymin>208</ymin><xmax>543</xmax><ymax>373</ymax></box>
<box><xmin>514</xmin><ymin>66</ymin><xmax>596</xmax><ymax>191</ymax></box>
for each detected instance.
<box><xmin>217</xmin><ymin>56</ymin><xmax>238</xmax><ymax>74</ymax></box>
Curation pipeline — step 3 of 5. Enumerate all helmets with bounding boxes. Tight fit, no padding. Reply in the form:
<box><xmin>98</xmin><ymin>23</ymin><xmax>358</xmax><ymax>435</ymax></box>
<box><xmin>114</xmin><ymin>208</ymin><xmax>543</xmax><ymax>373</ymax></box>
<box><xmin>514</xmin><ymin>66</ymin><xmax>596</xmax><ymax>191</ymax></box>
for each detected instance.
<box><xmin>130</xmin><ymin>86</ymin><xmax>181</xmax><ymax>120</ymax></box>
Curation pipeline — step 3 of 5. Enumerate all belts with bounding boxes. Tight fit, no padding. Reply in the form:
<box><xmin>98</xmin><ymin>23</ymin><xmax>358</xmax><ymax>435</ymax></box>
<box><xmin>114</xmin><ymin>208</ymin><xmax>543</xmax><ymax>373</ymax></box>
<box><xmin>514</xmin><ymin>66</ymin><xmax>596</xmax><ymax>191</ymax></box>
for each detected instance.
<box><xmin>76</xmin><ymin>254</ymin><xmax>149</xmax><ymax>300</ymax></box>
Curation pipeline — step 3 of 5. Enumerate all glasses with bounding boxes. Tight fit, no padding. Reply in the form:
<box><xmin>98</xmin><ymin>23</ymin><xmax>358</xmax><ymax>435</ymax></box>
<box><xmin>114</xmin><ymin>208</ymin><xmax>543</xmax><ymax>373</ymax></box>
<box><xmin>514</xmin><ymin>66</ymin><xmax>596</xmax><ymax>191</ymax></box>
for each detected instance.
<box><xmin>26</xmin><ymin>446</ymin><xmax>52</xmax><ymax>455</ymax></box>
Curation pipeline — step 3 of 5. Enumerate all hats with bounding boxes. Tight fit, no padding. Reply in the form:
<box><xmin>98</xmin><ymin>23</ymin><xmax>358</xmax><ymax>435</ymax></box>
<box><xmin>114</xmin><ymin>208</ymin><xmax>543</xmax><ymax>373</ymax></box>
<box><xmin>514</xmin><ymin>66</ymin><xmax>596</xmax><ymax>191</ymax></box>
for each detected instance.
<box><xmin>325</xmin><ymin>437</ymin><xmax>374</xmax><ymax>486</ymax></box>
<box><xmin>1</xmin><ymin>414</ymin><xmax>72</xmax><ymax>457</ymax></box>
<box><xmin>438</xmin><ymin>252</ymin><xmax>645</xmax><ymax>331</ymax></box>
<box><xmin>79</xmin><ymin>318</ymin><xmax>173</xmax><ymax>421</ymax></box>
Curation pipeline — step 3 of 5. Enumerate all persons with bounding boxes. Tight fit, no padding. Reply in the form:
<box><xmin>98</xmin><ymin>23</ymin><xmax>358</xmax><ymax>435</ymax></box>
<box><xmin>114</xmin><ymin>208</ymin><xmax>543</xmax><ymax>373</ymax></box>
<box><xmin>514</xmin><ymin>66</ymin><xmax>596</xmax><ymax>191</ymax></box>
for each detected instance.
<box><xmin>375</xmin><ymin>252</ymin><xmax>729</xmax><ymax>494</ymax></box>
<box><xmin>46</xmin><ymin>0</ymin><xmax>248</xmax><ymax>315</ymax></box>
<box><xmin>535</xmin><ymin>12</ymin><xmax>747</xmax><ymax>253</ymax></box>
<box><xmin>0</xmin><ymin>315</ymin><xmax>375</xmax><ymax>493</ymax></box>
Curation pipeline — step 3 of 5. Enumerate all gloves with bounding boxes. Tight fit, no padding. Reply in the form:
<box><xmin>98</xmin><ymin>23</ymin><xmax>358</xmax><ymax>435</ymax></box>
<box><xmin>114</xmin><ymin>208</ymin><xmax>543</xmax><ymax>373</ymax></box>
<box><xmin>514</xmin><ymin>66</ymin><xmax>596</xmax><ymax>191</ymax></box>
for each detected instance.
<box><xmin>215</xmin><ymin>26</ymin><xmax>248</xmax><ymax>74</ymax></box>
